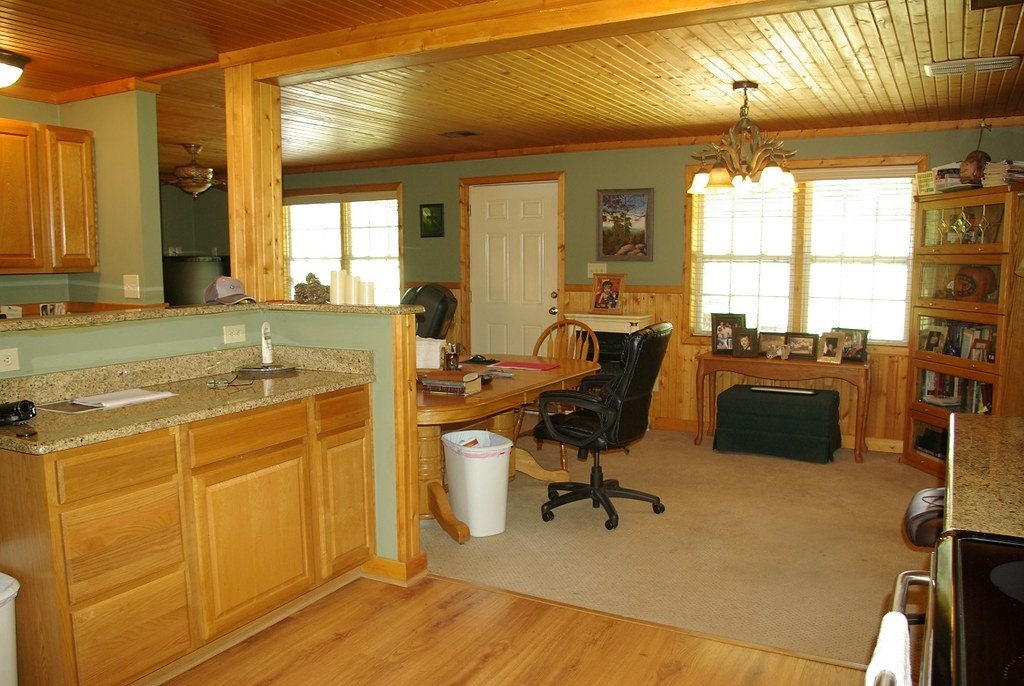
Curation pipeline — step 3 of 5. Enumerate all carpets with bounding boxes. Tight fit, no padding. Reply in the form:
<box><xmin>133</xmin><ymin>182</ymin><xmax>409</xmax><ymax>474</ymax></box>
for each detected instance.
<box><xmin>419</xmin><ymin>427</ymin><xmax>944</xmax><ymax>683</ymax></box>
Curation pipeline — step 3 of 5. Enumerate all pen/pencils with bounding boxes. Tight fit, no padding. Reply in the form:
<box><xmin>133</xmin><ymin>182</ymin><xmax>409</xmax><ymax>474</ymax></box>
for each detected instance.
<box><xmin>445</xmin><ymin>342</ymin><xmax>456</xmax><ymax>365</ymax></box>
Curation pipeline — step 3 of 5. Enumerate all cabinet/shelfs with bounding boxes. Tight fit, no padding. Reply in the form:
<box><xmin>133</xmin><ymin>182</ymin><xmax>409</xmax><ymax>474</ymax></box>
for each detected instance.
<box><xmin>898</xmin><ymin>183</ymin><xmax>1024</xmax><ymax>480</ymax></box>
<box><xmin>0</xmin><ymin>118</ymin><xmax>100</xmax><ymax>275</ymax></box>
<box><xmin>0</xmin><ymin>382</ymin><xmax>377</xmax><ymax>686</ymax></box>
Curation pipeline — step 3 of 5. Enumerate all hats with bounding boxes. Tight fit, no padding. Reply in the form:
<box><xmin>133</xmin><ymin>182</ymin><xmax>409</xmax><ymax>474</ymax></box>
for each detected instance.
<box><xmin>204</xmin><ymin>276</ymin><xmax>256</xmax><ymax>306</ymax></box>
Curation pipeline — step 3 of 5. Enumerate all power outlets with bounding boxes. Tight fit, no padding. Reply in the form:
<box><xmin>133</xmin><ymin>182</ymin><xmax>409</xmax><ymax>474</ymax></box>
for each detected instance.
<box><xmin>224</xmin><ymin>324</ymin><xmax>246</xmax><ymax>344</ymax></box>
<box><xmin>0</xmin><ymin>347</ymin><xmax>20</xmax><ymax>373</ymax></box>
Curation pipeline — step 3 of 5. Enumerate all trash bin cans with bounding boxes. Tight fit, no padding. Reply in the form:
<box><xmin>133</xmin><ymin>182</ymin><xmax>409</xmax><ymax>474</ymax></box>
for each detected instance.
<box><xmin>441</xmin><ymin>430</ymin><xmax>513</xmax><ymax>536</ymax></box>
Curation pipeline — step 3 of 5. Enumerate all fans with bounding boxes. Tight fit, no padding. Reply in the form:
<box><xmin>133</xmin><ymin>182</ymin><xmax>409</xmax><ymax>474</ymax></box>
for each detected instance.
<box><xmin>160</xmin><ymin>144</ymin><xmax>227</xmax><ymax>198</ymax></box>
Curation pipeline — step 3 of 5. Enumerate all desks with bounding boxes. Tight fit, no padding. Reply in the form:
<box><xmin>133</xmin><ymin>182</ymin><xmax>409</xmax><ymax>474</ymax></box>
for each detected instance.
<box><xmin>694</xmin><ymin>350</ymin><xmax>874</xmax><ymax>462</ymax></box>
<box><xmin>943</xmin><ymin>412</ymin><xmax>1024</xmax><ymax>539</ymax></box>
<box><xmin>415</xmin><ymin>353</ymin><xmax>602</xmax><ymax>545</ymax></box>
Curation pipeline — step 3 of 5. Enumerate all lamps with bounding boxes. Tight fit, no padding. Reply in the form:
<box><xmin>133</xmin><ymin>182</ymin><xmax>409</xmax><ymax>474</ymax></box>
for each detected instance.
<box><xmin>687</xmin><ymin>81</ymin><xmax>800</xmax><ymax>196</ymax></box>
<box><xmin>0</xmin><ymin>48</ymin><xmax>31</xmax><ymax>89</ymax></box>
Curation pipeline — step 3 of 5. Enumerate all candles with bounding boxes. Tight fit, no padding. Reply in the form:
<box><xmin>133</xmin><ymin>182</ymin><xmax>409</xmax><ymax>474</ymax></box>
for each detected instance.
<box><xmin>331</xmin><ymin>270</ymin><xmax>375</xmax><ymax>305</ymax></box>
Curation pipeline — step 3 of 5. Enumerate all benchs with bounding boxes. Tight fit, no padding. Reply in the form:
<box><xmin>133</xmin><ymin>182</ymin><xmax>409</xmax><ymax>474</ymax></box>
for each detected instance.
<box><xmin>712</xmin><ymin>384</ymin><xmax>843</xmax><ymax>464</ymax></box>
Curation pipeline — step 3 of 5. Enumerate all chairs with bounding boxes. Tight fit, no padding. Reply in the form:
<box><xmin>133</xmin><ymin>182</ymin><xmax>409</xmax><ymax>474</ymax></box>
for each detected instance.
<box><xmin>399</xmin><ymin>283</ymin><xmax>457</xmax><ymax>339</ymax></box>
<box><xmin>533</xmin><ymin>321</ymin><xmax>674</xmax><ymax>531</ymax></box>
<box><xmin>513</xmin><ymin>319</ymin><xmax>600</xmax><ymax>473</ymax></box>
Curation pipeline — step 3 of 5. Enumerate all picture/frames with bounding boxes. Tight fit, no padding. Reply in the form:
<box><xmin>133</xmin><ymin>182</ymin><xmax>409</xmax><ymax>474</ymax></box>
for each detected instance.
<box><xmin>711</xmin><ymin>313</ymin><xmax>870</xmax><ymax>363</ymax></box>
<box><xmin>587</xmin><ymin>273</ymin><xmax>626</xmax><ymax>315</ymax></box>
<box><xmin>419</xmin><ymin>203</ymin><xmax>444</xmax><ymax>238</ymax></box>
<box><xmin>923</xmin><ymin>324</ymin><xmax>948</xmax><ymax>354</ymax></box>
<box><xmin>597</xmin><ymin>187</ymin><xmax>655</xmax><ymax>262</ymax></box>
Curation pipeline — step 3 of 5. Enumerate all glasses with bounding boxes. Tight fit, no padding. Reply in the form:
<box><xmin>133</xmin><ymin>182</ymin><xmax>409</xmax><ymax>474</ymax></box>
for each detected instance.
<box><xmin>206</xmin><ymin>375</ymin><xmax>255</xmax><ymax>390</ymax></box>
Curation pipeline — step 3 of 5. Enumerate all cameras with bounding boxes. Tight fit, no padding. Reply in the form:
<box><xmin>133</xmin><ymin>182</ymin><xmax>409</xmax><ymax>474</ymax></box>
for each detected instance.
<box><xmin>0</xmin><ymin>400</ymin><xmax>37</xmax><ymax>426</ymax></box>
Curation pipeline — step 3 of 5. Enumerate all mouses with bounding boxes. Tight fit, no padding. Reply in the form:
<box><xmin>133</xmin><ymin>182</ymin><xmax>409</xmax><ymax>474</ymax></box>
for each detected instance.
<box><xmin>471</xmin><ymin>354</ymin><xmax>486</xmax><ymax>362</ymax></box>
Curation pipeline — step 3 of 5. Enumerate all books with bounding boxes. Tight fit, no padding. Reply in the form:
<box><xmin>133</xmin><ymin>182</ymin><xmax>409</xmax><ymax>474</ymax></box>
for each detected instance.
<box><xmin>422</xmin><ymin>370</ymin><xmax>482</xmax><ymax>395</ymax></box>
<box><xmin>933</xmin><ymin>161</ymin><xmax>982</xmax><ymax>193</ymax></box>
<box><xmin>921</xmin><ymin>320</ymin><xmax>999</xmax><ymax>415</ymax></box>
<box><xmin>981</xmin><ymin>160</ymin><xmax>1024</xmax><ymax>187</ymax></box>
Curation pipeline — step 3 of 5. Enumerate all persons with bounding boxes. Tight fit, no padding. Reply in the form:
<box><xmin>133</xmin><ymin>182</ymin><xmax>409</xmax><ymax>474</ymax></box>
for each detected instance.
<box><xmin>717</xmin><ymin>322</ymin><xmax>751</xmax><ymax>350</ymax></box>
<box><xmin>789</xmin><ymin>336</ymin><xmax>852</xmax><ymax>358</ymax></box>
